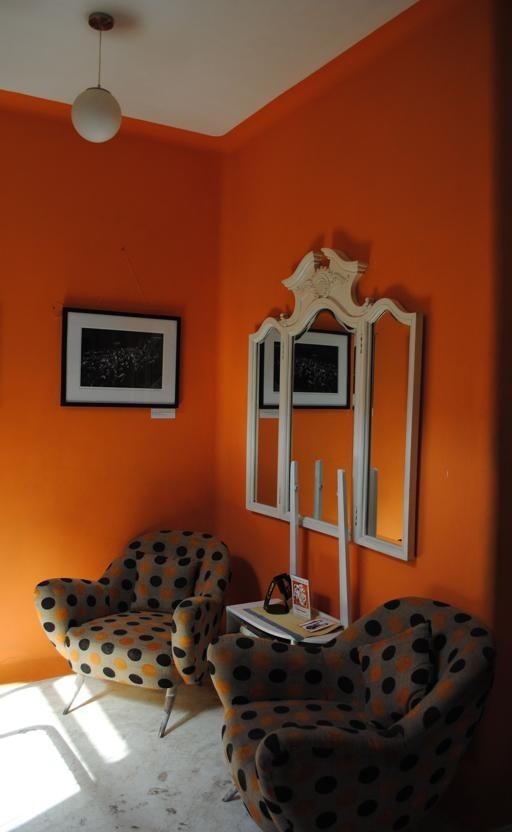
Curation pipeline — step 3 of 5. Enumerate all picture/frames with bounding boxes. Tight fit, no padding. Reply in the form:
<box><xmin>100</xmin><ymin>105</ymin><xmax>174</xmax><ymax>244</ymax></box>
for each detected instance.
<box><xmin>256</xmin><ymin>328</ymin><xmax>351</xmax><ymax>410</ymax></box>
<box><xmin>60</xmin><ymin>303</ymin><xmax>181</xmax><ymax>409</ymax></box>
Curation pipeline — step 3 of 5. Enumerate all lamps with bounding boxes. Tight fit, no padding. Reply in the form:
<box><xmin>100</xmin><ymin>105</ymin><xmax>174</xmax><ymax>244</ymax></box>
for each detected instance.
<box><xmin>70</xmin><ymin>12</ymin><xmax>122</xmax><ymax>145</ymax></box>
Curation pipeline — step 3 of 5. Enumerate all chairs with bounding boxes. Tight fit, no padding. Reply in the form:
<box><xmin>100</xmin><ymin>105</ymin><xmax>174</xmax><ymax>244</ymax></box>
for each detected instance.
<box><xmin>35</xmin><ymin>529</ymin><xmax>235</xmax><ymax>738</ymax></box>
<box><xmin>207</xmin><ymin>596</ymin><xmax>496</xmax><ymax>832</ymax></box>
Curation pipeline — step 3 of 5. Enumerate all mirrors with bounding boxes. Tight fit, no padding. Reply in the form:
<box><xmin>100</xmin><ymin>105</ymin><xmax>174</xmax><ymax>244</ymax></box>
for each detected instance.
<box><xmin>245</xmin><ymin>249</ymin><xmax>427</xmax><ymax>562</ymax></box>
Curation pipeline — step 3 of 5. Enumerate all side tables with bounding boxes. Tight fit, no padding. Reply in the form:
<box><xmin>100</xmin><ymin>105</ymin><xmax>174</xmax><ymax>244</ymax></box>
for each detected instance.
<box><xmin>225</xmin><ymin>597</ymin><xmax>345</xmax><ymax>647</ymax></box>
